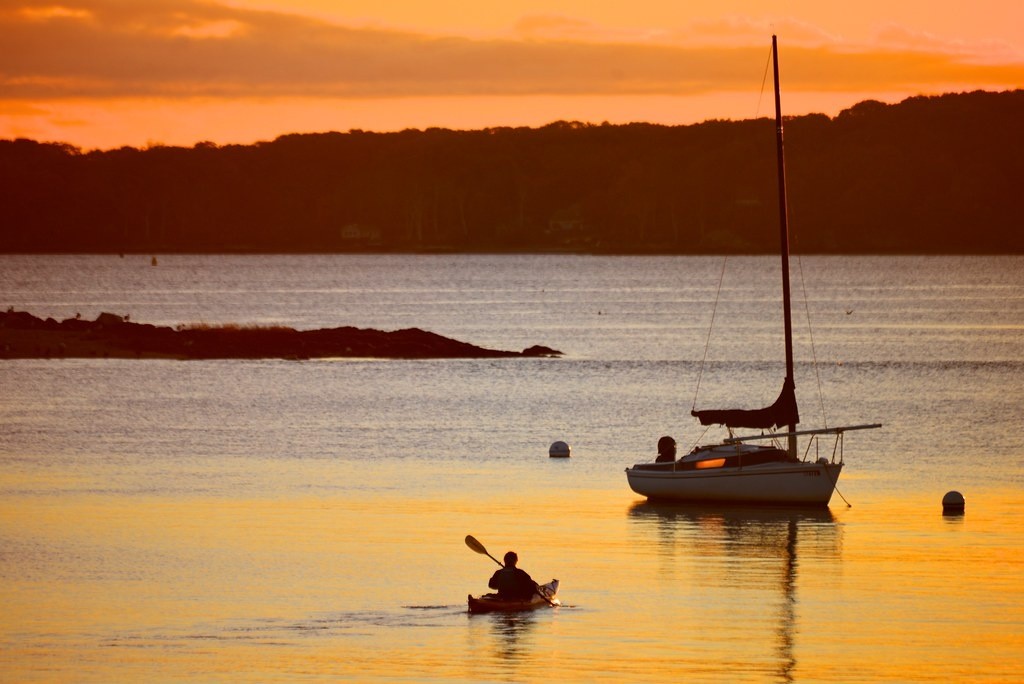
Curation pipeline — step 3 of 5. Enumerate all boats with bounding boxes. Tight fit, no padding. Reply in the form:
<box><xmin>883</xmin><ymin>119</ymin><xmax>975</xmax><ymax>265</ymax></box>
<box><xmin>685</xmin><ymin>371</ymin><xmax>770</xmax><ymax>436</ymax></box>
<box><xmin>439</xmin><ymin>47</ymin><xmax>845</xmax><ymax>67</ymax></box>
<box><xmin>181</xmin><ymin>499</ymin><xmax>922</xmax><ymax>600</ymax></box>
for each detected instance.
<box><xmin>468</xmin><ymin>578</ymin><xmax>560</xmax><ymax>616</ymax></box>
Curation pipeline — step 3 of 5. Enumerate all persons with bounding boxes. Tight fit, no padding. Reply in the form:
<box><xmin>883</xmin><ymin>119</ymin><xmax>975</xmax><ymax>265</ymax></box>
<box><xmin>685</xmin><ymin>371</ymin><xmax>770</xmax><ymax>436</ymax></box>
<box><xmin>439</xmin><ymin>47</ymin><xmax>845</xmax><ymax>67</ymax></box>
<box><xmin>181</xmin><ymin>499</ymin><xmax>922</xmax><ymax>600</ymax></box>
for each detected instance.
<box><xmin>488</xmin><ymin>551</ymin><xmax>536</xmax><ymax>598</ymax></box>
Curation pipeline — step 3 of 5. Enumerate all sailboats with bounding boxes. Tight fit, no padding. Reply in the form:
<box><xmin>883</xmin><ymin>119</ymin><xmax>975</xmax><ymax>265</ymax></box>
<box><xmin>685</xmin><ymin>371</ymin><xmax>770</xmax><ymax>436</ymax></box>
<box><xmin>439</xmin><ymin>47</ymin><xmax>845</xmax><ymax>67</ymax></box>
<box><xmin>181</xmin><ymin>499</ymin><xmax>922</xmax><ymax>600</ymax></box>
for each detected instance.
<box><xmin>624</xmin><ymin>33</ymin><xmax>884</xmax><ymax>511</ymax></box>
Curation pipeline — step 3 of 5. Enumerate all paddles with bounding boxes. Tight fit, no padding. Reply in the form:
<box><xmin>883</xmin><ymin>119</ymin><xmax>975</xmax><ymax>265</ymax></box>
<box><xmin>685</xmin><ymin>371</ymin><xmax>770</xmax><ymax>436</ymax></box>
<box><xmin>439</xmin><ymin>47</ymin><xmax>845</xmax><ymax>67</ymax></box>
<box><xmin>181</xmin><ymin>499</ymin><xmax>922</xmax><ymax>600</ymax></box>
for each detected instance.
<box><xmin>465</xmin><ymin>535</ymin><xmax>574</xmax><ymax>607</ymax></box>
